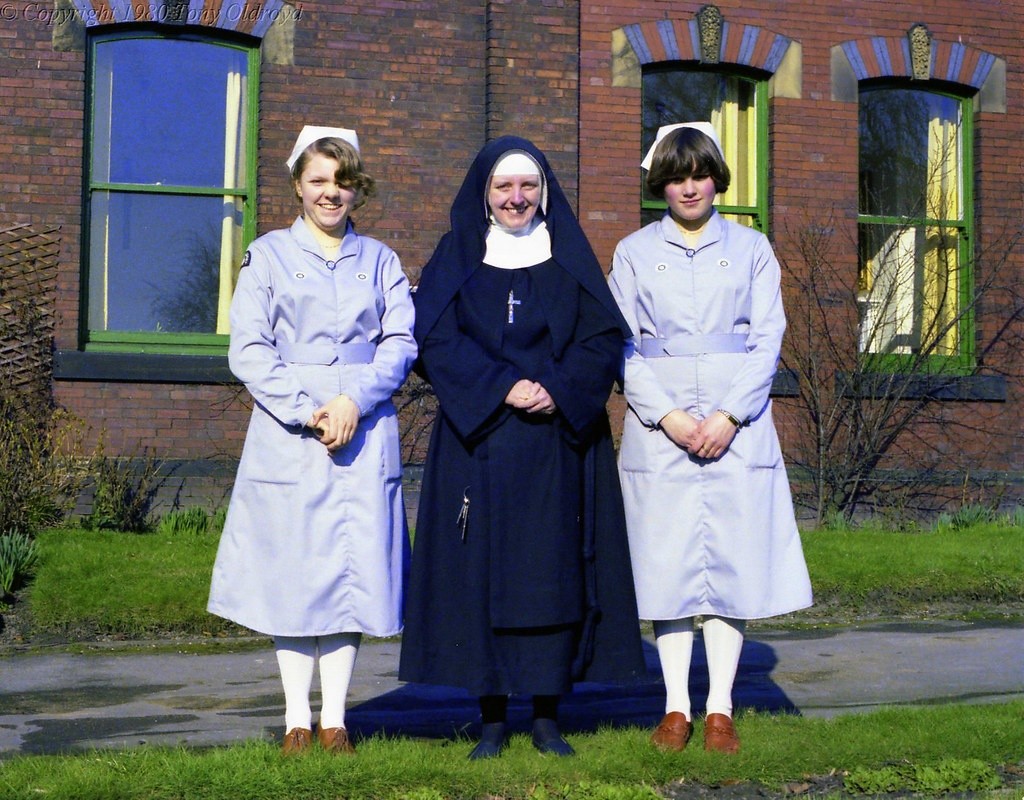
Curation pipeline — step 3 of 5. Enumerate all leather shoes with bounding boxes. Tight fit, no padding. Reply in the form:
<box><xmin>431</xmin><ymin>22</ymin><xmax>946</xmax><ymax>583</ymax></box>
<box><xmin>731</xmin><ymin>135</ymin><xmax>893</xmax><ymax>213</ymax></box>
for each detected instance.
<box><xmin>280</xmin><ymin>729</ymin><xmax>314</xmax><ymax>768</ymax></box>
<box><xmin>647</xmin><ymin>711</ymin><xmax>694</xmax><ymax>755</ymax></box>
<box><xmin>703</xmin><ymin>712</ymin><xmax>740</xmax><ymax>757</ymax></box>
<box><xmin>314</xmin><ymin>716</ymin><xmax>359</xmax><ymax>762</ymax></box>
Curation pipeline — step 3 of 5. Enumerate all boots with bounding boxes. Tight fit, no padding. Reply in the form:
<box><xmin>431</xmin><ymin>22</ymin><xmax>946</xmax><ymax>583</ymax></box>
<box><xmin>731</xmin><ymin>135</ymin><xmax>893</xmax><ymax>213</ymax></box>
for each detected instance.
<box><xmin>530</xmin><ymin>698</ymin><xmax>576</xmax><ymax>762</ymax></box>
<box><xmin>466</xmin><ymin>693</ymin><xmax>511</xmax><ymax>764</ymax></box>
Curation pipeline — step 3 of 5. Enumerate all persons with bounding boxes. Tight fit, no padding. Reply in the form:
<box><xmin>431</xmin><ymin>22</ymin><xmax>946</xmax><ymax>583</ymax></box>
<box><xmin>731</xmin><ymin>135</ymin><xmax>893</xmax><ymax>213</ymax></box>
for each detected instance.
<box><xmin>411</xmin><ymin>134</ymin><xmax>645</xmax><ymax>762</ymax></box>
<box><xmin>606</xmin><ymin>121</ymin><xmax>815</xmax><ymax>754</ymax></box>
<box><xmin>206</xmin><ymin>125</ymin><xmax>419</xmax><ymax>757</ymax></box>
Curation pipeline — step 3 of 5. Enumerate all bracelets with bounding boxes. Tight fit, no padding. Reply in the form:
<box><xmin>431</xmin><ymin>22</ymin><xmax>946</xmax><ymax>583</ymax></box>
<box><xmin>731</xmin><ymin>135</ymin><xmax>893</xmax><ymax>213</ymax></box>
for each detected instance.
<box><xmin>717</xmin><ymin>409</ymin><xmax>741</xmax><ymax>429</ymax></box>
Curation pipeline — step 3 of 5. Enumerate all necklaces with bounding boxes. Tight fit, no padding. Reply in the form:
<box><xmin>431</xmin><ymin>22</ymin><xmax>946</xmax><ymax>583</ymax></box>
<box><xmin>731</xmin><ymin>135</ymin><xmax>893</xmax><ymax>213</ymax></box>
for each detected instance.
<box><xmin>506</xmin><ymin>290</ymin><xmax>522</xmax><ymax>324</ymax></box>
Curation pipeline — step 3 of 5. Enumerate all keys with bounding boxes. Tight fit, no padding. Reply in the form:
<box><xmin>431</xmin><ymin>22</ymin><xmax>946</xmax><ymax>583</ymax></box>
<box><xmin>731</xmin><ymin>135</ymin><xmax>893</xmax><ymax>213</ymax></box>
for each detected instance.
<box><xmin>456</xmin><ymin>497</ymin><xmax>469</xmax><ymax>540</ymax></box>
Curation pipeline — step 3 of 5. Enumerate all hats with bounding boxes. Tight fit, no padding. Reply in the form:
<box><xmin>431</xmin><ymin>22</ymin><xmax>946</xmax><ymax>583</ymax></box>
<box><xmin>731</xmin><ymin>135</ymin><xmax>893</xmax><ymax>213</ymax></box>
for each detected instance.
<box><xmin>285</xmin><ymin>124</ymin><xmax>362</xmax><ymax>174</ymax></box>
<box><xmin>639</xmin><ymin>122</ymin><xmax>727</xmax><ymax>171</ymax></box>
